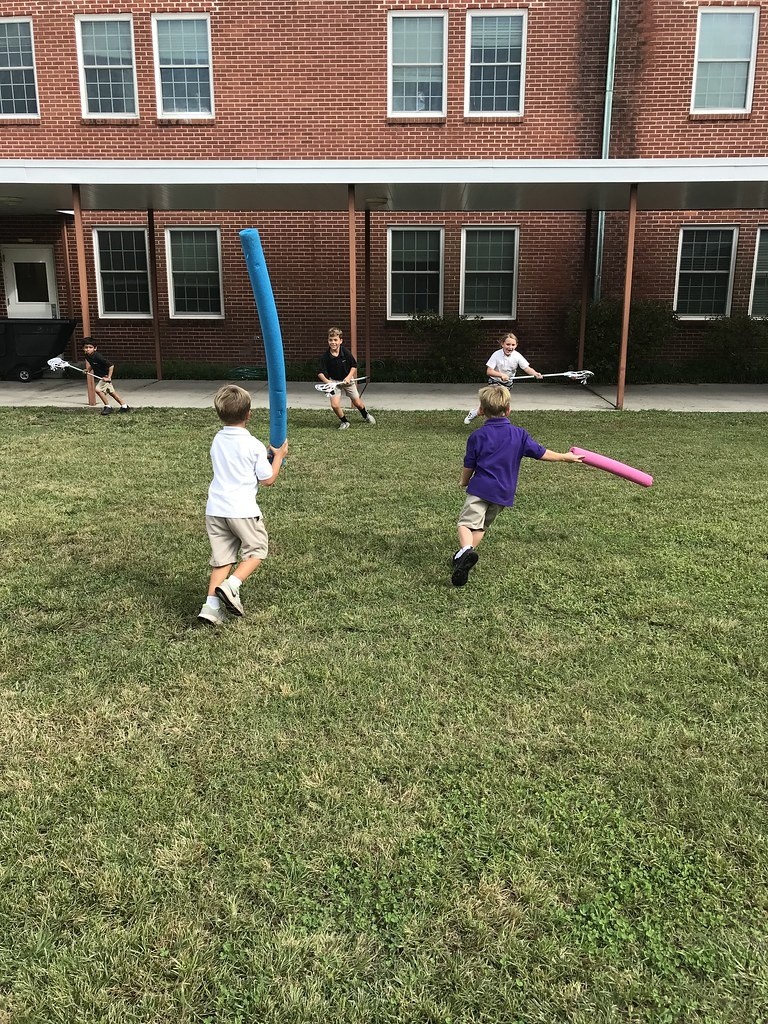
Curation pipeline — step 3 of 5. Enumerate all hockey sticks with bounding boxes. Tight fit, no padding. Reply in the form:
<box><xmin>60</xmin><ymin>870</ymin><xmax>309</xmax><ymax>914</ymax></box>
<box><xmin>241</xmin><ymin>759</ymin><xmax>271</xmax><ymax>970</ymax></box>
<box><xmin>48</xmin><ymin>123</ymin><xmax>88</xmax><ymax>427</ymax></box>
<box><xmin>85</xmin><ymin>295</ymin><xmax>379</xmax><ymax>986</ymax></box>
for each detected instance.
<box><xmin>509</xmin><ymin>370</ymin><xmax>595</xmax><ymax>379</ymax></box>
<box><xmin>47</xmin><ymin>358</ymin><xmax>112</xmax><ymax>384</ymax></box>
<box><xmin>314</xmin><ymin>375</ymin><xmax>370</xmax><ymax>393</ymax></box>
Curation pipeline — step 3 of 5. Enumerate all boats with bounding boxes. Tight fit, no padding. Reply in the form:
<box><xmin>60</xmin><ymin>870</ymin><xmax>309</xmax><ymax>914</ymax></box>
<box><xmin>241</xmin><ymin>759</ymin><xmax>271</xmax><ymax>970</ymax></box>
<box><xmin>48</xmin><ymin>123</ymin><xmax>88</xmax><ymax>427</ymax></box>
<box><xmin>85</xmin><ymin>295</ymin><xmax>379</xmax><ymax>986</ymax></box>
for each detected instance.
<box><xmin>0</xmin><ymin>318</ymin><xmax>79</xmax><ymax>380</ymax></box>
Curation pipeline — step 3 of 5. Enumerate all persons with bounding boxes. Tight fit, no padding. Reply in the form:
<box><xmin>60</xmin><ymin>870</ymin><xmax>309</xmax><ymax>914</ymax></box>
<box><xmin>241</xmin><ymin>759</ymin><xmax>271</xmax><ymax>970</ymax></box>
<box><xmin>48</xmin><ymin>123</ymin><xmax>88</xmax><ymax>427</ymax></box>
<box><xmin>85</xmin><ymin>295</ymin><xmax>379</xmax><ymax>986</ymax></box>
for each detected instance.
<box><xmin>81</xmin><ymin>337</ymin><xmax>130</xmax><ymax>415</ymax></box>
<box><xmin>452</xmin><ymin>384</ymin><xmax>586</xmax><ymax>587</ymax></box>
<box><xmin>317</xmin><ymin>326</ymin><xmax>377</xmax><ymax>430</ymax></box>
<box><xmin>463</xmin><ymin>332</ymin><xmax>544</xmax><ymax>425</ymax></box>
<box><xmin>197</xmin><ymin>385</ymin><xmax>288</xmax><ymax>627</ymax></box>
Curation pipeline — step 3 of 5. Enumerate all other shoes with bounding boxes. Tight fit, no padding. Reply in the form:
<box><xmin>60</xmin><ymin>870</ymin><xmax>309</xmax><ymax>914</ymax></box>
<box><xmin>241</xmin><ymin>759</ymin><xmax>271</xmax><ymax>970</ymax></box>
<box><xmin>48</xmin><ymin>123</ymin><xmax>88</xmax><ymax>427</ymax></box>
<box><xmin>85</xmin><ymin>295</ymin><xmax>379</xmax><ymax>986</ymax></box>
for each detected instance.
<box><xmin>452</xmin><ymin>550</ymin><xmax>461</xmax><ymax>565</ymax></box>
<box><xmin>452</xmin><ymin>547</ymin><xmax>478</xmax><ymax>586</ymax></box>
<box><xmin>117</xmin><ymin>405</ymin><xmax>130</xmax><ymax>415</ymax></box>
<box><xmin>100</xmin><ymin>406</ymin><xmax>114</xmax><ymax>415</ymax></box>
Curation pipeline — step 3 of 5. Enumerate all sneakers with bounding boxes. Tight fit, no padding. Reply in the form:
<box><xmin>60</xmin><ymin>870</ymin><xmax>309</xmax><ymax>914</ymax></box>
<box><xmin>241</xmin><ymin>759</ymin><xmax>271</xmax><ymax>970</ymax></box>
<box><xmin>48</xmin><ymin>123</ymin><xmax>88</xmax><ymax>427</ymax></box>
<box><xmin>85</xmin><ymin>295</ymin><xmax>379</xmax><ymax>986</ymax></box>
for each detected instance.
<box><xmin>215</xmin><ymin>579</ymin><xmax>244</xmax><ymax>616</ymax></box>
<box><xmin>365</xmin><ymin>412</ymin><xmax>376</xmax><ymax>425</ymax></box>
<box><xmin>338</xmin><ymin>422</ymin><xmax>351</xmax><ymax>430</ymax></box>
<box><xmin>464</xmin><ymin>409</ymin><xmax>476</xmax><ymax>425</ymax></box>
<box><xmin>197</xmin><ymin>604</ymin><xmax>230</xmax><ymax>627</ymax></box>
